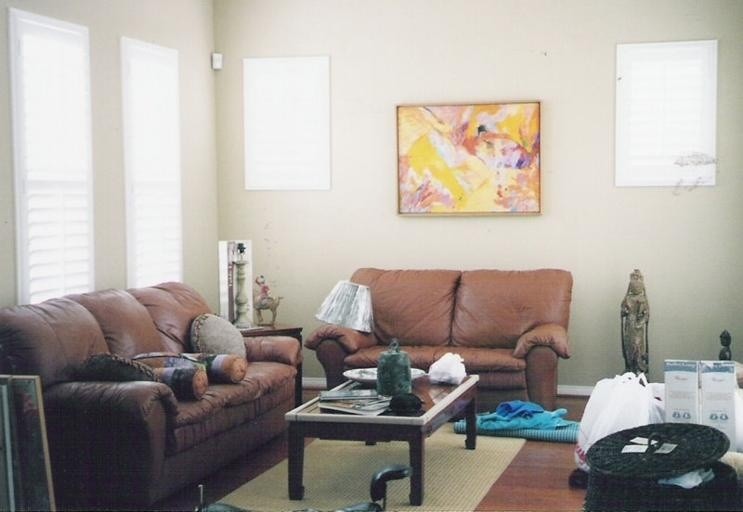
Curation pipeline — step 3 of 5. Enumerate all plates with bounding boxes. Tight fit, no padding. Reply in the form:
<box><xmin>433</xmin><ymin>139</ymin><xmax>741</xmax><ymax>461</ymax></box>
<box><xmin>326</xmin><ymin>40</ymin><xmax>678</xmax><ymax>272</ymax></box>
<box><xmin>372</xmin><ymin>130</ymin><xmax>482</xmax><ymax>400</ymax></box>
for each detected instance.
<box><xmin>342</xmin><ymin>367</ymin><xmax>426</xmax><ymax>387</ymax></box>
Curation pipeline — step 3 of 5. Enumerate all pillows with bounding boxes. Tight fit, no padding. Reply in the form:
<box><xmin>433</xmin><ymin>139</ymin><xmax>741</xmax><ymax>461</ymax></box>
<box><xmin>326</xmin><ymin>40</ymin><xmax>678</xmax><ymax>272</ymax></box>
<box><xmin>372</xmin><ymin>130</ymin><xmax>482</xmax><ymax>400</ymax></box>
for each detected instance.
<box><xmin>316</xmin><ymin>278</ymin><xmax>375</xmax><ymax>334</ymax></box>
<box><xmin>77</xmin><ymin>354</ymin><xmax>156</xmax><ymax>382</ymax></box>
<box><xmin>136</xmin><ymin>348</ymin><xmax>208</xmax><ymax>399</ymax></box>
<box><xmin>189</xmin><ymin>309</ymin><xmax>248</xmax><ymax>361</ymax></box>
<box><xmin>184</xmin><ymin>350</ymin><xmax>246</xmax><ymax>384</ymax></box>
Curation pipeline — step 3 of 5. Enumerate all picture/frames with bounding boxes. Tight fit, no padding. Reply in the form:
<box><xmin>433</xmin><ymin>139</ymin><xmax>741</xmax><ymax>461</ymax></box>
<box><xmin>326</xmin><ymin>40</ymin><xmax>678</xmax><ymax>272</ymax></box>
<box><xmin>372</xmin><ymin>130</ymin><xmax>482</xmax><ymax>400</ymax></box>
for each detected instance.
<box><xmin>614</xmin><ymin>42</ymin><xmax>715</xmax><ymax>188</ymax></box>
<box><xmin>244</xmin><ymin>60</ymin><xmax>332</xmax><ymax>191</ymax></box>
<box><xmin>397</xmin><ymin>102</ymin><xmax>543</xmax><ymax>214</ymax></box>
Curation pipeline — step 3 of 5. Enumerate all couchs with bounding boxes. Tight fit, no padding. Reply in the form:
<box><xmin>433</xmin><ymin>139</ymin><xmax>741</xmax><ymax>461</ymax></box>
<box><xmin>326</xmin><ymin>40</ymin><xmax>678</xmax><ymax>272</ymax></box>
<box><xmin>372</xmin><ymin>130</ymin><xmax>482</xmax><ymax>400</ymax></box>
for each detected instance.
<box><xmin>2</xmin><ymin>285</ymin><xmax>301</xmax><ymax>505</ymax></box>
<box><xmin>307</xmin><ymin>266</ymin><xmax>571</xmax><ymax>415</ymax></box>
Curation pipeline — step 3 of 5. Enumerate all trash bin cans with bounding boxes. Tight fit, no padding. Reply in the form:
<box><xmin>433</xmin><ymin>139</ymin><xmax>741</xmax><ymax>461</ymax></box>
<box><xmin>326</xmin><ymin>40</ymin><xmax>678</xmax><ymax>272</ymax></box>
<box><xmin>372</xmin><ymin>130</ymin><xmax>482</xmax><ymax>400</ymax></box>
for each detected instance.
<box><xmin>580</xmin><ymin>423</ymin><xmax>743</xmax><ymax>512</ymax></box>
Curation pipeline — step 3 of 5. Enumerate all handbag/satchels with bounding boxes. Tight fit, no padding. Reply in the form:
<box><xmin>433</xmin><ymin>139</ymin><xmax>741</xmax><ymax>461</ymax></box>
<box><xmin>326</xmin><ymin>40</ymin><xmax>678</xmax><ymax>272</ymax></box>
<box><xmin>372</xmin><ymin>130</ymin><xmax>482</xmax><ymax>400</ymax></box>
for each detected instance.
<box><xmin>575</xmin><ymin>372</ymin><xmax>664</xmax><ymax>473</ymax></box>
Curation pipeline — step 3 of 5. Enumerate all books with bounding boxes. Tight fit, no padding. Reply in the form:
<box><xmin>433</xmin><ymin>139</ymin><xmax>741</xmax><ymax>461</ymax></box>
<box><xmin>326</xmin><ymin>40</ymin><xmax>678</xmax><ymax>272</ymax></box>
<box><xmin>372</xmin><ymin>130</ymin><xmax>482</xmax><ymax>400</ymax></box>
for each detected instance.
<box><xmin>316</xmin><ymin>385</ymin><xmax>391</xmax><ymax>420</ymax></box>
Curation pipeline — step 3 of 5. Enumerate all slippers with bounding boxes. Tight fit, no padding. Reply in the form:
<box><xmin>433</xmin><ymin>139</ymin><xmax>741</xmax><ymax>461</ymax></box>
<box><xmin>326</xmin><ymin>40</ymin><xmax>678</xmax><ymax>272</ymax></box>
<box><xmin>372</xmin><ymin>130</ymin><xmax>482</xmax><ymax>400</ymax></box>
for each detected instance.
<box><xmin>569</xmin><ymin>468</ymin><xmax>588</xmax><ymax>489</ymax></box>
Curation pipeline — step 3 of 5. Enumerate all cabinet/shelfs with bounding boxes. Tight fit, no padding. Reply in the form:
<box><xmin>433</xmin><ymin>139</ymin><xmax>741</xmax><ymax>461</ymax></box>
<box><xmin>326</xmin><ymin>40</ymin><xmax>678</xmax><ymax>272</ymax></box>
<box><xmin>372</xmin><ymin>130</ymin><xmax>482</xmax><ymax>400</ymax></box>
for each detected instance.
<box><xmin>237</xmin><ymin>320</ymin><xmax>302</xmax><ymax>409</ymax></box>
